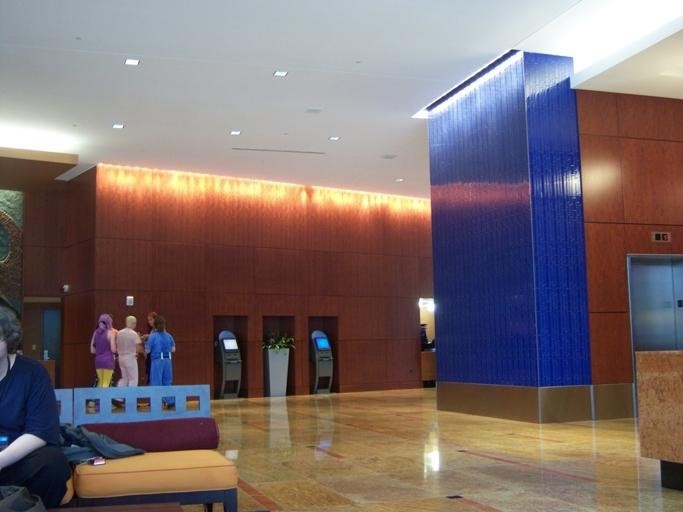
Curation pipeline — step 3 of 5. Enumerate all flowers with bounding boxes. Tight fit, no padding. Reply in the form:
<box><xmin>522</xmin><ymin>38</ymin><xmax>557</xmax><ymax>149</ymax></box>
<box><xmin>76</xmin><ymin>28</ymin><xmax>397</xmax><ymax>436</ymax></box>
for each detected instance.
<box><xmin>261</xmin><ymin>328</ymin><xmax>296</xmax><ymax>350</ymax></box>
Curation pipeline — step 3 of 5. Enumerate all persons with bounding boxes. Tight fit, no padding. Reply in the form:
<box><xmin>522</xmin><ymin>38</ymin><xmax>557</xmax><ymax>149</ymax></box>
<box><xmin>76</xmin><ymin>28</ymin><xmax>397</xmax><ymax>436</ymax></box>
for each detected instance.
<box><xmin>0</xmin><ymin>306</ymin><xmax>72</xmax><ymax>512</ymax></box>
<box><xmin>85</xmin><ymin>310</ymin><xmax>176</xmax><ymax>408</ymax></box>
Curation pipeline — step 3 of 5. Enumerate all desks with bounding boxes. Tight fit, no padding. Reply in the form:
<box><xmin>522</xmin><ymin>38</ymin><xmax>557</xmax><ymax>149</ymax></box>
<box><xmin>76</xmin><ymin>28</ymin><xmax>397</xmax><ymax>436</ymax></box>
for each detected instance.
<box><xmin>36</xmin><ymin>359</ymin><xmax>56</xmax><ymax>389</ymax></box>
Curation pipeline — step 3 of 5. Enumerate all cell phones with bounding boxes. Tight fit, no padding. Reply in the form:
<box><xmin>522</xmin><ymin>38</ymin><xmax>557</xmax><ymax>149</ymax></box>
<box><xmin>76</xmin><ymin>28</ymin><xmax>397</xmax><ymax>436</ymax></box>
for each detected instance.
<box><xmin>92</xmin><ymin>457</ymin><xmax>106</xmax><ymax>465</ymax></box>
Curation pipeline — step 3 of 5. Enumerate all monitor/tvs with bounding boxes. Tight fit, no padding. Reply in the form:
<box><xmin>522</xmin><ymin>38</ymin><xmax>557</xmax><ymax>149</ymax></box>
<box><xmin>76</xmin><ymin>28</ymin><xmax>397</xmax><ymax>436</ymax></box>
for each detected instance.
<box><xmin>223</xmin><ymin>338</ymin><xmax>239</xmax><ymax>350</ymax></box>
<box><xmin>314</xmin><ymin>337</ymin><xmax>330</xmax><ymax>350</ymax></box>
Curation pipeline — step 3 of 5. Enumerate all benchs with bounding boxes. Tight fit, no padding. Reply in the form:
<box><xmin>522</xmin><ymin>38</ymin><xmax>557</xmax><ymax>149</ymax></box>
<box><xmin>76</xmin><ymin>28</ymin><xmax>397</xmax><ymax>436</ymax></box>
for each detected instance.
<box><xmin>0</xmin><ymin>384</ymin><xmax>240</xmax><ymax>512</ymax></box>
